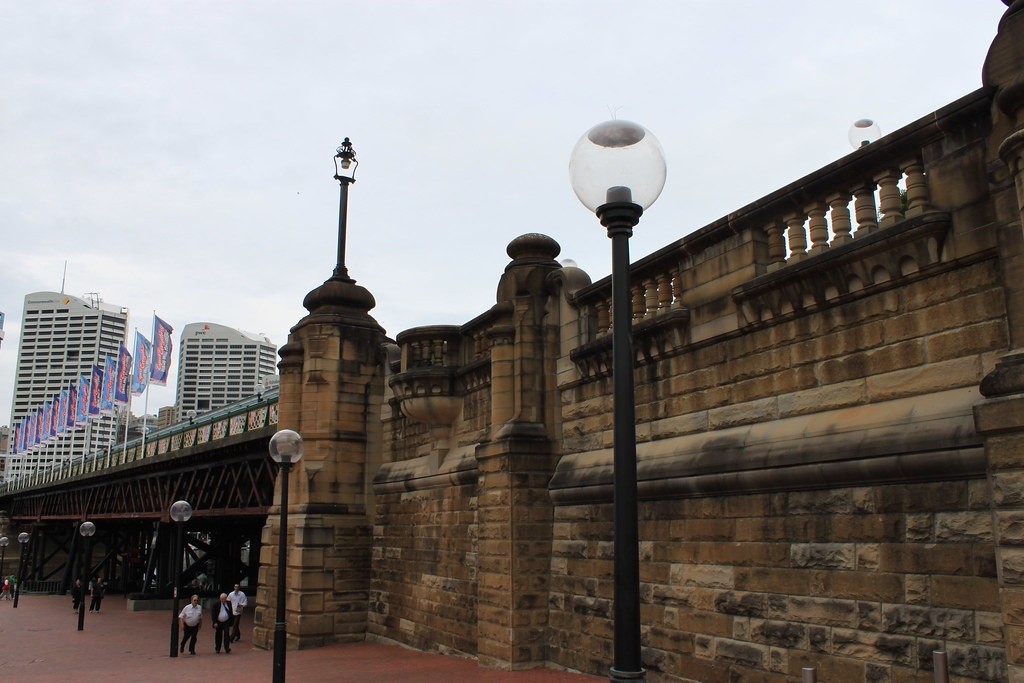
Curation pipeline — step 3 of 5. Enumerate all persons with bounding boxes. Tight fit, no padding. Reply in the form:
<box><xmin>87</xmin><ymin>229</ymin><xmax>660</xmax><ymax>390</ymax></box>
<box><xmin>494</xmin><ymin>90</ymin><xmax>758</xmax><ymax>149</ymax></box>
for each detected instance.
<box><xmin>212</xmin><ymin>593</ymin><xmax>233</xmax><ymax>654</ymax></box>
<box><xmin>0</xmin><ymin>574</ymin><xmax>15</xmax><ymax>600</ymax></box>
<box><xmin>227</xmin><ymin>584</ymin><xmax>248</xmax><ymax>643</ymax></box>
<box><xmin>89</xmin><ymin>577</ymin><xmax>104</xmax><ymax>614</ymax></box>
<box><xmin>178</xmin><ymin>594</ymin><xmax>203</xmax><ymax>655</ymax></box>
<box><xmin>71</xmin><ymin>578</ymin><xmax>82</xmax><ymax>614</ymax></box>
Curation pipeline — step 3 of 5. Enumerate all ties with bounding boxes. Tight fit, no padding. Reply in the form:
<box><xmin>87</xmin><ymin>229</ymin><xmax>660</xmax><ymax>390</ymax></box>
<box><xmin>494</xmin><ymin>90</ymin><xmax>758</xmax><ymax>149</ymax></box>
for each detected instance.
<box><xmin>223</xmin><ymin>603</ymin><xmax>231</xmax><ymax>617</ymax></box>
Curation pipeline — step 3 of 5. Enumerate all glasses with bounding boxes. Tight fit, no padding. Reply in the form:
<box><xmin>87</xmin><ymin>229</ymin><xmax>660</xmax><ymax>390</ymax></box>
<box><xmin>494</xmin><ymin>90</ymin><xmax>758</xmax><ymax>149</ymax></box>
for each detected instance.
<box><xmin>194</xmin><ymin>599</ymin><xmax>198</xmax><ymax>602</ymax></box>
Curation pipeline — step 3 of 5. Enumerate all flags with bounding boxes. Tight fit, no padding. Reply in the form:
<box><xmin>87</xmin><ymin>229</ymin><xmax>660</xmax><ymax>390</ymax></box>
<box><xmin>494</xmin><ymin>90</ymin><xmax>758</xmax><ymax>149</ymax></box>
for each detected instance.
<box><xmin>13</xmin><ymin>314</ymin><xmax>174</xmax><ymax>456</ymax></box>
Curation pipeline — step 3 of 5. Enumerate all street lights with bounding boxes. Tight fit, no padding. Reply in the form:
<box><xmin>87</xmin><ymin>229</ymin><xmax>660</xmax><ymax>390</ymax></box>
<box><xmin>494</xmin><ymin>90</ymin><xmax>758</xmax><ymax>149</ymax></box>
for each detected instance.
<box><xmin>560</xmin><ymin>257</ymin><xmax>576</xmax><ymax>268</ymax></box>
<box><xmin>569</xmin><ymin>119</ymin><xmax>667</xmax><ymax>683</ymax></box>
<box><xmin>267</xmin><ymin>428</ymin><xmax>305</xmax><ymax>682</ymax></box>
<box><xmin>323</xmin><ymin>137</ymin><xmax>359</xmax><ymax>283</ymax></box>
<box><xmin>168</xmin><ymin>499</ymin><xmax>193</xmax><ymax>656</ymax></box>
<box><xmin>77</xmin><ymin>521</ymin><xmax>96</xmax><ymax>632</ymax></box>
<box><xmin>13</xmin><ymin>532</ymin><xmax>30</xmax><ymax>608</ymax></box>
<box><xmin>0</xmin><ymin>536</ymin><xmax>9</xmax><ymax>576</ymax></box>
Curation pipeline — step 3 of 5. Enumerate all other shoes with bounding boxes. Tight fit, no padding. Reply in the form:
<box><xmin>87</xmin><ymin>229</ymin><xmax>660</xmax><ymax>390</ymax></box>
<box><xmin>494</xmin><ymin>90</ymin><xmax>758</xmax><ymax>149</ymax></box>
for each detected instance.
<box><xmin>226</xmin><ymin>648</ymin><xmax>231</xmax><ymax>653</ymax></box>
<box><xmin>229</xmin><ymin>636</ymin><xmax>240</xmax><ymax>643</ymax></box>
<box><xmin>189</xmin><ymin>649</ymin><xmax>196</xmax><ymax>655</ymax></box>
<box><xmin>180</xmin><ymin>647</ymin><xmax>184</xmax><ymax>652</ymax></box>
<box><xmin>217</xmin><ymin>648</ymin><xmax>220</xmax><ymax>653</ymax></box>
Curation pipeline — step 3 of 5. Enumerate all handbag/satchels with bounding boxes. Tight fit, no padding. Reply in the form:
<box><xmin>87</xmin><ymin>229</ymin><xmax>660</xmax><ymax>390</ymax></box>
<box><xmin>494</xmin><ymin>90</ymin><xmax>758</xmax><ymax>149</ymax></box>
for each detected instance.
<box><xmin>0</xmin><ymin>581</ymin><xmax>4</xmax><ymax>588</ymax></box>
<box><xmin>100</xmin><ymin>592</ymin><xmax>104</xmax><ymax>599</ymax></box>
